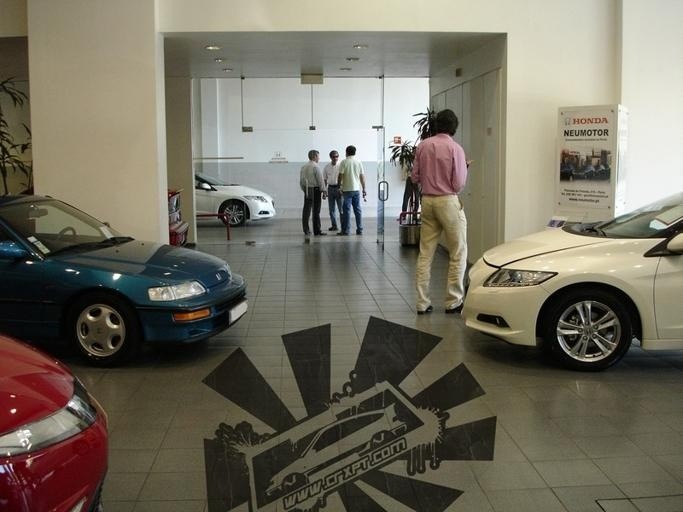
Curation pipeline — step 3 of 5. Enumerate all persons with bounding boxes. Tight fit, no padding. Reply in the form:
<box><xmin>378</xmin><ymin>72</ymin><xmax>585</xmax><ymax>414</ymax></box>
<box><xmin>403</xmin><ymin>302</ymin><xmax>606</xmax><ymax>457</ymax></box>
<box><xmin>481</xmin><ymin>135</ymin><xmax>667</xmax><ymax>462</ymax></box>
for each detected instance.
<box><xmin>396</xmin><ymin>146</ymin><xmax>420</xmax><ymax>221</ymax></box>
<box><xmin>337</xmin><ymin>145</ymin><xmax>367</xmax><ymax>235</ymax></box>
<box><xmin>411</xmin><ymin>109</ymin><xmax>469</xmax><ymax>314</ymax></box>
<box><xmin>300</xmin><ymin>149</ymin><xmax>327</xmax><ymax>235</ymax></box>
<box><xmin>323</xmin><ymin>151</ymin><xmax>344</xmax><ymax>231</ymax></box>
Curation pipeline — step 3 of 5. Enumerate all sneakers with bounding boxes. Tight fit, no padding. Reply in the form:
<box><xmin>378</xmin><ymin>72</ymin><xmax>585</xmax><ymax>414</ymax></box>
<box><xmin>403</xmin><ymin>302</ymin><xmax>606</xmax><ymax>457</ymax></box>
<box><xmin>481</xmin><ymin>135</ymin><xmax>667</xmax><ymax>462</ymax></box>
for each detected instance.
<box><xmin>445</xmin><ymin>302</ymin><xmax>463</xmax><ymax>314</ymax></box>
<box><xmin>418</xmin><ymin>305</ymin><xmax>432</xmax><ymax>314</ymax></box>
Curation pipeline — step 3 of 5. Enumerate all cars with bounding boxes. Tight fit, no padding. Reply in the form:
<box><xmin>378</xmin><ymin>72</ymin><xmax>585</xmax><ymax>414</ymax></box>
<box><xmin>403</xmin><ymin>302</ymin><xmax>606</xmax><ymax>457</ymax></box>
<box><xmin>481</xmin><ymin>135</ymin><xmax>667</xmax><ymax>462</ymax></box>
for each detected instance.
<box><xmin>0</xmin><ymin>193</ymin><xmax>249</xmax><ymax>367</ymax></box>
<box><xmin>459</xmin><ymin>192</ymin><xmax>683</xmax><ymax>373</ymax></box>
<box><xmin>0</xmin><ymin>185</ymin><xmax>189</xmax><ymax>248</ymax></box>
<box><xmin>195</xmin><ymin>172</ymin><xmax>276</xmax><ymax>227</ymax></box>
<box><xmin>0</xmin><ymin>332</ymin><xmax>111</xmax><ymax>511</ymax></box>
<box><xmin>262</xmin><ymin>402</ymin><xmax>408</xmax><ymax>499</ymax></box>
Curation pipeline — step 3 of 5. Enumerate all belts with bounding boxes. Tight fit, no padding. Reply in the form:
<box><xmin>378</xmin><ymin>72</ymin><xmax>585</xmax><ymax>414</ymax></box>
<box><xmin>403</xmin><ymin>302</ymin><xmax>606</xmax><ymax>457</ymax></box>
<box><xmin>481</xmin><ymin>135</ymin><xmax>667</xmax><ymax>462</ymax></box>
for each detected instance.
<box><xmin>329</xmin><ymin>184</ymin><xmax>340</xmax><ymax>187</ymax></box>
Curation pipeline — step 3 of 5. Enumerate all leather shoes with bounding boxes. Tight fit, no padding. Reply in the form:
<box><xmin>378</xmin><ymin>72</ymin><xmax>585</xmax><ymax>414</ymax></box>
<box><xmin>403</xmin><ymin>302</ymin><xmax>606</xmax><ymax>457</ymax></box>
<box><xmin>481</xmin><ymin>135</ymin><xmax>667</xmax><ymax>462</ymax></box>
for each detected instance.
<box><xmin>305</xmin><ymin>231</ymin><xmax>310</xmax><ymax>234</ymax></box>
<box><xmin>357</xmin><ymin>230</ymin><xmax>362</xmax><ymax>234</ymax></box>
<box><xmin>314</xmin><ymin>230</ymin><xmax>327</xmax><ymax>235</ymax></box>
<box><xmin>337</xmin><ymin>232</ymin><xmax>348</xmax><ymax>235</ymax></box>
<box><xmin>329</xmin><ymin>226</ymin><xmax>337</xmax><ymax>230</ymax></box>
